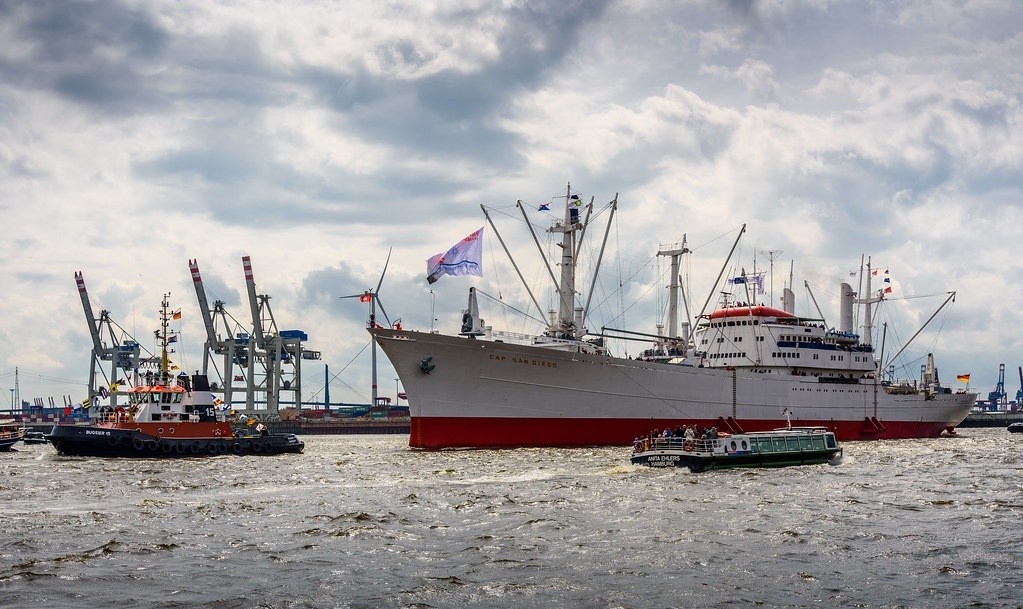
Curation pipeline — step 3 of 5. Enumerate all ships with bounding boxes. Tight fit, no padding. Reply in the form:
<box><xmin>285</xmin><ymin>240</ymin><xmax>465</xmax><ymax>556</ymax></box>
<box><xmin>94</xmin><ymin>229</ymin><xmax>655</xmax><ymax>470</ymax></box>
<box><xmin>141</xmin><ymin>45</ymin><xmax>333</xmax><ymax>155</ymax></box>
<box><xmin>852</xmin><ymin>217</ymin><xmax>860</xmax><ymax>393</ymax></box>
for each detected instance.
<box><xmin>366</xmin><ymin>180</ymin><xmax>979</xmax><ymax>450</ymax></box>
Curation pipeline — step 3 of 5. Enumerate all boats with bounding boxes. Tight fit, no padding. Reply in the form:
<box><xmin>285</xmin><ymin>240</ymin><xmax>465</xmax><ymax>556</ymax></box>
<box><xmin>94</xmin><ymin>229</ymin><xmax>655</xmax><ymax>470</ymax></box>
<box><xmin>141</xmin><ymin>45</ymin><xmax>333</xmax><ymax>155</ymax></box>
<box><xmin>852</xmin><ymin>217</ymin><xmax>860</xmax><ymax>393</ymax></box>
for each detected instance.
<box><xmin>957</xmin><ymin>411</ymin><xmax>1023</xmax><ymax>427</ymax></box>
<box><xmin>631</xmin><ymin>402</ymin><xmax>843</xmax><ymax>474</ymax></box>
<box><xmin>1007</xmin><ymin>423</ymin><xmax>1023</xmax><ymax>433</ymax></box>
<box><xmin>46</xmin><ymin>291</ymin><xmax>305</xmax><ymax>459</ymax></box>
<box><xmin>0</xmin><ymin>422</ymin><xmax>51</xmax><ymax>452</ymax></box>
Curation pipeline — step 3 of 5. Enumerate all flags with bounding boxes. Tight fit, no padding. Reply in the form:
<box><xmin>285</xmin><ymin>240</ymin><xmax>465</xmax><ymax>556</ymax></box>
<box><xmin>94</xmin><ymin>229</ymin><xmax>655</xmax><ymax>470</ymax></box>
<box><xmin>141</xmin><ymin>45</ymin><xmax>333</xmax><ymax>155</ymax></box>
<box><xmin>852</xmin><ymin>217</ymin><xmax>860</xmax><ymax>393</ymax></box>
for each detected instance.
<box><xmin>568</xmin><ymin>192</ymin><xmax>584</xmax><ymax>225</ymax></box>
<box><xmin>848</xmin><ymin>269</ymin><xmax>892</xmax><ymax>293</ymax></box>
<box><xmin>536</xmin><ymin>201</ymin><xmax>551</xmax><ymax>212</ymax></box>
<box><xmin>957</xmin><ymin>373</ymin><xmax>971</xmax><ymax>382</ymax></box>
<box><xmin>173</xmin><ymin>311</ymin><xmax>182</xmax><ymax>320</ymax></box>
<box><xmin>728</xmin><ymin>274</ymin><xmax>765</xmax><ymax>295</ymax></box>
<box><xmin>167</xmin><ymin>335</ymin><xmax>178</xmax><ymax>343</ymax></box>
<box><xmin>424</xmin><ymin>227</ymin><xmax>483</xmax><ymax>286</ymax></box>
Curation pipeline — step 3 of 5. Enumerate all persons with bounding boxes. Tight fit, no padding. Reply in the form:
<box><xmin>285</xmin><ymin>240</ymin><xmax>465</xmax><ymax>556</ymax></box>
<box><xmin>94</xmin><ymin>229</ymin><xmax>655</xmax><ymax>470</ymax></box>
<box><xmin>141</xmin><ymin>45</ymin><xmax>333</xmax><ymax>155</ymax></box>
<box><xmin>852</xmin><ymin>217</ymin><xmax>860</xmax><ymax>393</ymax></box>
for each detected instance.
<box><xmin>630</xmin><ymin>422</ymin><xmax>719</xmax><ymax>453</ymax></box>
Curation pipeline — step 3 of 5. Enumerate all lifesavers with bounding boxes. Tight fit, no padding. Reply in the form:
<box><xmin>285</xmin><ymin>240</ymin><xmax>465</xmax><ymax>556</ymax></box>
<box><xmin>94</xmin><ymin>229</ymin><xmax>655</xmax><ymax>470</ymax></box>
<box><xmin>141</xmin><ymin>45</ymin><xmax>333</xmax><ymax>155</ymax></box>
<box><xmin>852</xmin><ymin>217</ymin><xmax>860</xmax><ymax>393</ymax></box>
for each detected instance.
<box><xmin>636</xmin><ymin>442</ymin><xmax>644</xmax><ymax>453</ymax></box>
<box><xmin>682</xmin><ymin>440</ymin><xmax>694</xmax><ymax>452</ymax></box>
